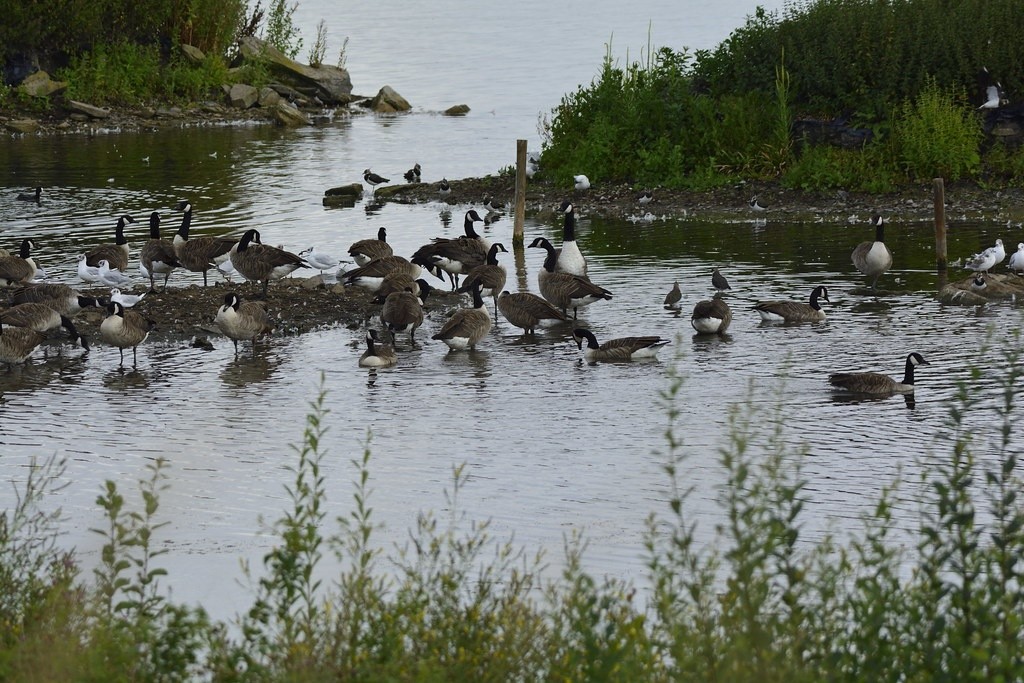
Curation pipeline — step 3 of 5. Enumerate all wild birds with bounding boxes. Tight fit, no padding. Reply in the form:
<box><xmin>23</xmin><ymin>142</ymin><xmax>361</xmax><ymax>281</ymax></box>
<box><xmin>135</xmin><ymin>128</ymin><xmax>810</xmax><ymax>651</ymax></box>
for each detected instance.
<box><xmin>361</xmin><ymin>168</ymin><xmax>390</xmax><ymax>192</ymax></box>
<box><xmin>15</xmin><ymin>186</ymin><xmax>44</xmax><ymax>203</ymax></box>
<box><xmin>97</xmin><ymin>259</ymin><xmax>136</xmax><ymax>291</ymax></box>
<box><xmin>0</xmin><ymin>213</ymin><xmax>158</xmax><ymax>371</ymax></box>
<box><xmin>975</xmin><ymin>84</ymin><xmax>1010</xmax><ymax>110</ymax></box>
<box><xmin>848</xmin><ymin>214</ymin><xmax>896</xmax><ymax>289</ymax></box>
<box><xmin>403</xmin><ymin>162</ymin><xmax>422</xmax><ymax>184</ymax></box>
<box><xmin>72</xmin><ymin>252</ymin><xmax>118</xmax><ymax>289</ymax></box>
<box><xmin>298</xmin><ymin>247</ymin><xmax>354</xmax><ymax>276</ymax></box>
<box><xmin>748</xmin><ymin>193</ymin><xmax>770</xmax><ymax>213</ymax></box>
<box><xmin>664</xmin><ymin>282</ymin><xmax>681</xmax><ymax>310</ymax></box>
<box><xmin>137</xmin><ymin>200</ymin><xmax>313</xmax><ymax>361</ymax></box>
<box><xmin>437</xmin><ymin>177</ymin><xmax>452</xmax><ymax>201</ymax></box>
<box><xmin>572</xmin><ymin>174</ymin><xmax>592</xmax><ymax>196</ymax></box>
<box><xmin>826</xmin><ymin>351</ymin><xmax>931</xmax><ymax>395</ymax></box>
<box><xmin>346</xmin><ymin>201</ymin><xmax>833</xmax><ymax>369</ymax></box>
<box><xmin>709</xmin><ymin>266</ymin><xmax>731</xmax><ymax>295</ymax></box>
<box><xmin>275</xmin><ymin>243</ymin><xmax>292</xmax><ymax>280</ymax></box>
<box><xmin>957</xmin><ymin>235</ymin><xmax>1024</xmax><ymax>292</ymax></box>
<box><xmin>213</xmin><ymin>257</ymin><xmax>237</xmax><ymax>284</ymax></box>
